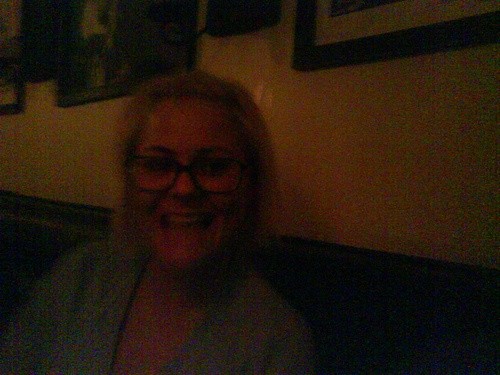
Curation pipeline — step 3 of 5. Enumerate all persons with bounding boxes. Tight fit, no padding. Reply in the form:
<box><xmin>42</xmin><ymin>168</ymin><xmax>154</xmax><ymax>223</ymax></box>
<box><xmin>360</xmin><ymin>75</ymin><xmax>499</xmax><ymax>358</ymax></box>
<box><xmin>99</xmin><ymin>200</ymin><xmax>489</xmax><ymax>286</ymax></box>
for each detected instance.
<box><xmin>1</xmin><ymin>69</ymin><xmax>317</xmax><ymax>374</ymax></box>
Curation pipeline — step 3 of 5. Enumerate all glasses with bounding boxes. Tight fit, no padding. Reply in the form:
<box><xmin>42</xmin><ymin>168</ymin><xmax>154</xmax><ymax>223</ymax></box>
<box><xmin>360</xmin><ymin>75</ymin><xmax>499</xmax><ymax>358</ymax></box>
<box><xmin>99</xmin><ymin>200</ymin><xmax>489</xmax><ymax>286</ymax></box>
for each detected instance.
<box><xmin>123</xmin><ymin>154</ymin><xmax>252</xmax><ymax>197</ymax></box>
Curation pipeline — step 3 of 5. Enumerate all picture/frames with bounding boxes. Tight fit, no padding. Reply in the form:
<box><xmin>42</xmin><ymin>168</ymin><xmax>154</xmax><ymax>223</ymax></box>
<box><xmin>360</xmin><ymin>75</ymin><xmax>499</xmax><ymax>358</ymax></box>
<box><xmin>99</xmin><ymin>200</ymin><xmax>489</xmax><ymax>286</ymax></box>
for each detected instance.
<box><xmin>290</xmin><ymin>1</ymin><xmax>500</xmax><ymax>72</ymax></box>
<box><xmin>52</xmin><ymin>1</ymin><xmax>202</xmax><ymax>106</ymax></box>
